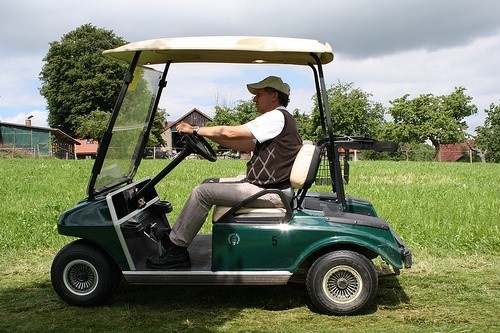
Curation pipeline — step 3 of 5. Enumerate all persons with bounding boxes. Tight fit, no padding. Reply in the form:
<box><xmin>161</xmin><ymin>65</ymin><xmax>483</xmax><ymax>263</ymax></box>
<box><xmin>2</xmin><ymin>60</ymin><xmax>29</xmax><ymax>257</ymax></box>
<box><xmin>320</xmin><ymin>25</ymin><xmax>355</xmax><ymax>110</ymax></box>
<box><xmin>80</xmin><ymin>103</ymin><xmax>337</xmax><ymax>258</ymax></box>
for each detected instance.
<box><xmin>146</xmin><ymin>76</ymin><xmax>303</xmax><ymax>270</ymax></box>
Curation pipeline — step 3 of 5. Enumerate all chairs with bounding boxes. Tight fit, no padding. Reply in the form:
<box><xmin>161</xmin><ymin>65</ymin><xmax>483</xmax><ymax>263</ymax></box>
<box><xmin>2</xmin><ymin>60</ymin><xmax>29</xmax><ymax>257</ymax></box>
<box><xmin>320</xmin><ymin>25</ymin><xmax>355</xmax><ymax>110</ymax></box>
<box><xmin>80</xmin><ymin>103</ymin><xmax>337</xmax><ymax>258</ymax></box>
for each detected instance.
<box><xmin>211</xmin><ymin>142</ymin><xmax>321</xmax><ymax>223</ymax></box>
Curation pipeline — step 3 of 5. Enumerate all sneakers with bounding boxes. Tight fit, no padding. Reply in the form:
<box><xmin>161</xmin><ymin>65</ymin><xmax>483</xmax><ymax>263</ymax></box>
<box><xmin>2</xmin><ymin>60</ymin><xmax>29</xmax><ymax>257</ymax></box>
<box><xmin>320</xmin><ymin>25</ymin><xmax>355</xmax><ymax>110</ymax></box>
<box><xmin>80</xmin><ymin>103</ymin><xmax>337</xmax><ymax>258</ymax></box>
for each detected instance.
<box><xmin>146</xmin><ymin>252</ymin><xmax>192</xmax><ymax>269</ymax></box>
<box><xmin>260</xmin><ymin>179</ymin><xmax>291</xmax><ymax>190</ymax></box>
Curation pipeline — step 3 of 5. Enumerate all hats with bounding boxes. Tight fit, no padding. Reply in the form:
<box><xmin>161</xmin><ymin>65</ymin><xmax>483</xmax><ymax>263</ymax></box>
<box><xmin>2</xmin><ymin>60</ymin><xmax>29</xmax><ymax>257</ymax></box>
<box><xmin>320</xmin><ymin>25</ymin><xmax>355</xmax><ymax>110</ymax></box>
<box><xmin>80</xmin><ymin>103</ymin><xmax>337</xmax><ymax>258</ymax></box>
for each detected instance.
<box><xmin>247</xmin><ymin>76</ymin><xmax>290</xmax><ymax>96</ymax></box>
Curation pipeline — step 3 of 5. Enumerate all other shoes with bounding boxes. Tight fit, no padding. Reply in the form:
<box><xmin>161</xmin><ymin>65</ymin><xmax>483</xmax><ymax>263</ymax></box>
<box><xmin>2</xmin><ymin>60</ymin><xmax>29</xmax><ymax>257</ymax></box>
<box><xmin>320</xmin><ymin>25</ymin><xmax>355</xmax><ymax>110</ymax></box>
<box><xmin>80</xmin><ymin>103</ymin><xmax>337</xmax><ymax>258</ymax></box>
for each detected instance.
<box><xmin>161</xmin><ymin>234</ymin><xmax>172</xmax><ymax>249</ymax></box>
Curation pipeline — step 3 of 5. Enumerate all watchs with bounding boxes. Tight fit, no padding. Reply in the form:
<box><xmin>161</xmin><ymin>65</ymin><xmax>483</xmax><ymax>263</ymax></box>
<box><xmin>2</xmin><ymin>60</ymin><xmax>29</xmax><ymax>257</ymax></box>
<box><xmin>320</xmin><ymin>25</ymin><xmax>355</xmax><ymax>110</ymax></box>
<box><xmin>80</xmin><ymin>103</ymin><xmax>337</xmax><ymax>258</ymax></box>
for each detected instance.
<box><xmin>192</xmin><ymin>125</ymin><xmax>200</xmax><ymax>135</ymax></box>
